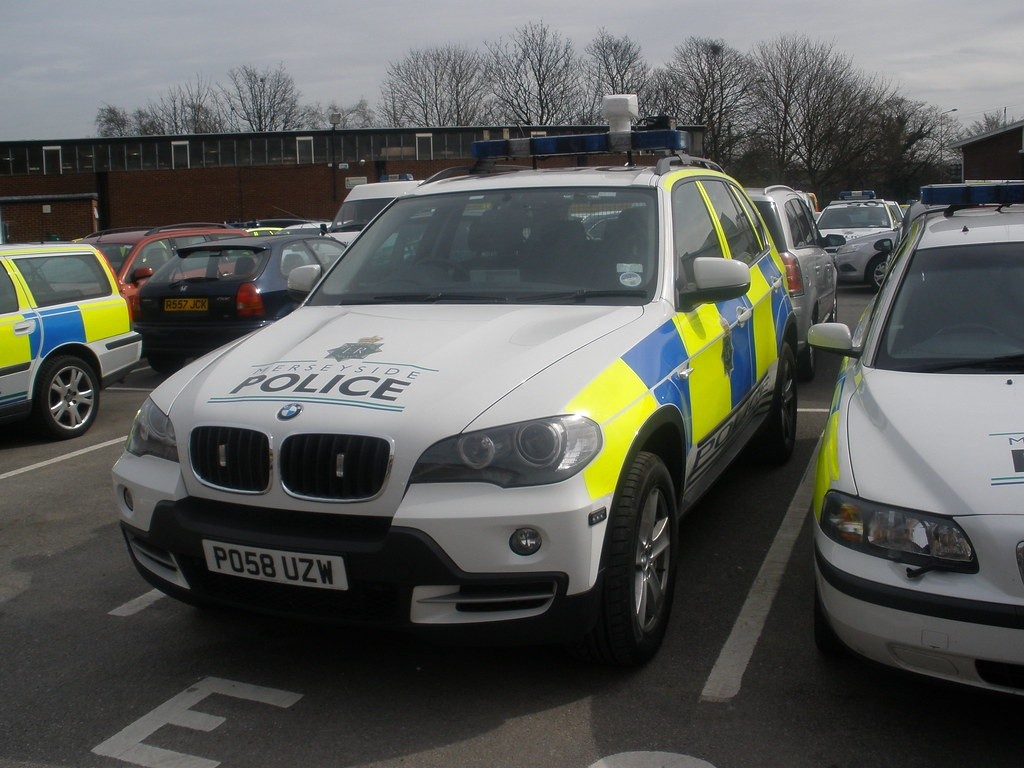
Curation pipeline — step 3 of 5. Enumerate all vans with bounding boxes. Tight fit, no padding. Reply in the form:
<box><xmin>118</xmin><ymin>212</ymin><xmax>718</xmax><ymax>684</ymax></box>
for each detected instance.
<box><xmin>313</xmin><ymin>179</ymin><xmax>434</xmax><ymax>270</ymax></box>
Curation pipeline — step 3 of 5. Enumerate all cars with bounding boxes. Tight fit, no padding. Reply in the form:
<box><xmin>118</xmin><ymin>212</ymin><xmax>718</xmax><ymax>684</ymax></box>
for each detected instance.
<box><xmin>808</xmin><ymin>203</ymin><xmax>1024</xmax><ymax>700</ymax></box>
<box><xmin>570</xmin><ymin>210</ymin><xmax>620</xmax><ymax>242</ymax></box>
<box><xmin>245</xmin><ymin>223</ymin><xmax>332</xmax><ymax>236</ymax></box>
<box><xmin>807</xmin><ymin>199</ymin><xmax>910</xmax><ymax>261</ymax></box>
<box><xmin>133</xmin><ymin>233</ymin><xmax>347</xmax><ymax>374</ymax></box>
<box><xmin>834</xmin><ymin>223</ymin><xmax>902</xmax><ymax>293</ymax></box>
<box><xmin>0</xmin><ymin>242</ymin><xmax>143</xmax><ymax>440</ymax></box>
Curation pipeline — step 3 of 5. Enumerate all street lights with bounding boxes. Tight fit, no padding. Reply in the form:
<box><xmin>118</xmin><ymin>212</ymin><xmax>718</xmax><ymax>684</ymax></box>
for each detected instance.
<box><xmin>330</xmin><ymin>112</ymin><xmax>341</xmax><ymax>202</ymax></box>
<box><xmin>940</xmin><ymin>109</ymin><xmax>957</xmax><ymax>184</ymax></box>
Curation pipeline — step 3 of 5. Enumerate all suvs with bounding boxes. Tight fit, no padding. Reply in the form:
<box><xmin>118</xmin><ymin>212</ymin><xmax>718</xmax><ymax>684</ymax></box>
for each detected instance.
<box><xmin>742</xmin><ymin>185</ymin><xmax>846</xmax><ymax>383</ymax></box>
<box><xmin>109</xmin><ymin>154</ymin><xmax>800</xmax><ymax>667</ymax></box>
<box><xmin>25</xmin><ymin>221</ymin><xmax>261</xmax><ymax>320</ymax></box>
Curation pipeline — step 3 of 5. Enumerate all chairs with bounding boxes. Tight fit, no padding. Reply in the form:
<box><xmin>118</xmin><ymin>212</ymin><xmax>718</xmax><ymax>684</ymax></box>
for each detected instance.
<box><xmin>553</xmin><ymin>221</ymin><xmax>592</xmax><ymax>243</ymax></box>
<box><xmin>825</xmin><ymin>214</ymin><xmax>851</xmax><ymax>227</ymax></box>
<box><xmin>447</xmin><ymin>207</ymin><xmax>539</xmax><ymax>284</ymax></box>
<box><xmin>600</xmin><ymin>204</ymin><xmax>688</xmax><ymax>289</ymax></box>
<box><xmin>282</xmin><ymin>254</ymin><xmax>305</xmax><ymax>273</ymax></box>
<box><xmin>234</xmin><ymin>255</ymin><xmax>256</xmax><ymax>275</ymax></box>
<box><xmin>145</xmin><ymin>248</ymin><xmax>168</xmax><ymax>272</ymax></box>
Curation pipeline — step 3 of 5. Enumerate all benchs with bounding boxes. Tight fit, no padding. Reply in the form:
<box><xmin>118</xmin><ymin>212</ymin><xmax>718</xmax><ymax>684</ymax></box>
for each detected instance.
<box><xmin>379</xmin><ymin>147</ymin><xmax>415</xmax><ymax>160</ymax></box>
